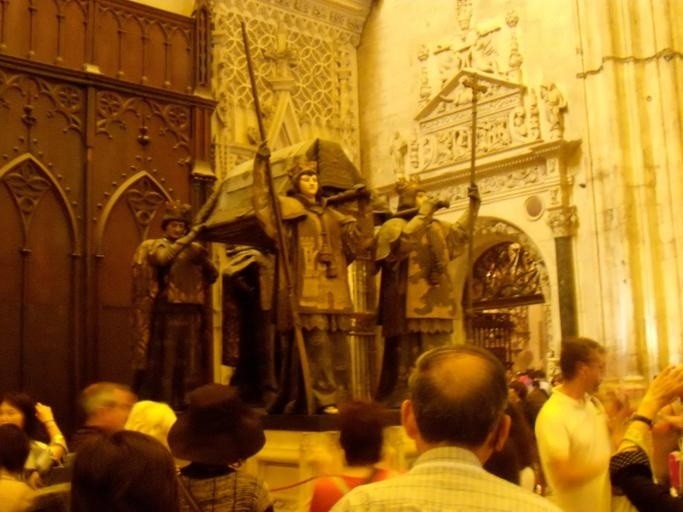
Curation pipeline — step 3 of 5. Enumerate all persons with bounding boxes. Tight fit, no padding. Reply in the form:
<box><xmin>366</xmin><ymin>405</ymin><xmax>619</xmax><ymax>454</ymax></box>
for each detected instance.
<box><xmin>309</xmin><ymin>411</ymin><xmax>400</xmax><ymax>512</ymax></box>
<box><xmin>251</xmin><ymin>138</ymin><xmax>375</xmax><ymax>415</ymax></box>
<box><xmin>542</xmin><ymin>80</ymin><xmax>567</xmax><ymax>142</ymax></box>
<box><xmin>372</xmin><ymin>178</ymin><xmax>483</xmax><ymax>409</ymax></box>
<box><xmin>482</xmin><ymin>338</ymin><xmax>683</xmax><ymax>511</ymax></box>
<box><xmin>129</xmin><ymin>176</ymin><xmax>233</xmax><ymax>412</ymax></box>
<box><xmin>1</xmin><ymin>379</ymin><xmax>288</xmax><ymax>512</ymax></box>
<box><xmin>388</xmin><ymin>131</ymin><xmax>409</xmax><ymax>182</ymax></box>
<box><xmin>327</xmin><ymin>343</ymin><xmax>561</xmax><ymax>512</ymax></box>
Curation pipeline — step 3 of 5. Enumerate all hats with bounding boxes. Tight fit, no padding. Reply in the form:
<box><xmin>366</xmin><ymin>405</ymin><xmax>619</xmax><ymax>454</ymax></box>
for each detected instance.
<box><xmin>168</xmin><ymin>383</ymin><xmax>266</xmax><ymax>464</ymax></box>
